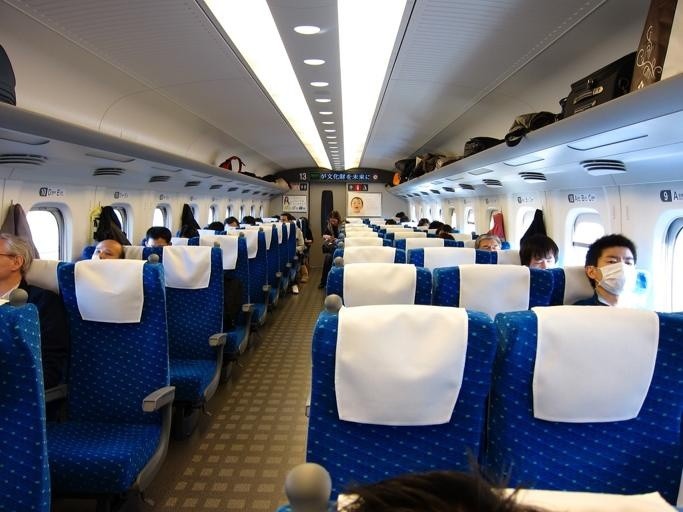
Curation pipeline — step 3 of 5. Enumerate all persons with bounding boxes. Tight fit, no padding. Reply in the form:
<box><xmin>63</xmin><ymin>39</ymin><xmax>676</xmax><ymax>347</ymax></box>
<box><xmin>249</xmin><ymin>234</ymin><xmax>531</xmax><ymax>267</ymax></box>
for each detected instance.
<box><xmin>519</xmin><ymin>234</ymin><xmax>559</xmax><ymax>270</ymax></box>
<box><xmin>0</xmin><ymin>232</ymin><xmax>65</xmax><ymax>413</ymax></box>
<box><xmin>91</xmin><ymin>238</ymin><xmax>123</xmax><ymax>261</ymax></box>
<box><xmin>283</xmin><ymin>196</ymin><xmax>290</xmax><ymax>208</ymax></box>
<box><xmin>339</xmin><ymin>469</ymin><xmax>555</xmax><ymax>512</ymax></box>
<box><xmin>145</xmin><ymin>212</ymin><xmax>313</xmax><ymax>343</ymax></box>
<box><xmin>570</xmin><ymin>234</ymin><xmax>641</xmax><ymax>306</ymax></box>
<box><xmin>350</xmin><ymin>197</ymin><xmax>363</xmax><ymax>213</ymax></box>
<box><xmin>317</xmin><ymin>209</ymin><xmax>350</xmax><ymax>288</ymax></box>
<box><xmin>385</xmin><ymin>210</ymin><xmax>501</xmax><ymax>250</ymax></box>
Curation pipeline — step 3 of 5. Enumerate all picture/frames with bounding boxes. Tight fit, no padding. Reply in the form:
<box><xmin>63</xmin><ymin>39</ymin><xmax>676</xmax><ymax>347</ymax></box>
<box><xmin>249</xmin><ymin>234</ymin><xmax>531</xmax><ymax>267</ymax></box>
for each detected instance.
<box><xmin>282</xmin><ymin>195</ymin><xmax>308</xmax><ymax>213</ymax></box>
<box><xmin>346</xmin><ymin>192</ymin><xmax>383</xmax><ymax>218</ymax></box>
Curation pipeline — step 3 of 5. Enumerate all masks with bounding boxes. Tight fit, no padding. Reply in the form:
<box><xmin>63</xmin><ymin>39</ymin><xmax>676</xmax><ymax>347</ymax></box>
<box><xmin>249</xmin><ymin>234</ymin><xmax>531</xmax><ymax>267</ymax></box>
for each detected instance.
<box><xmin>585</xmin><ymin>261</ymin><xmax>637</xmax><ymax>297</ymax></box>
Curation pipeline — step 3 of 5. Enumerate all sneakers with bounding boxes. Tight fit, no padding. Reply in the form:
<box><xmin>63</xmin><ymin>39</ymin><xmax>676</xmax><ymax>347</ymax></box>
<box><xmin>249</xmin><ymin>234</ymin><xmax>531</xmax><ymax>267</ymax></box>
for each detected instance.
<box><xmin>301</xmin><ymin>275</ymin><xmax>309</xmax><ymax>283</ymax></box>
<box><xmin>292</xmin><ymin>285</ymin><xmax>299</xmax><ymax>293</ymax></box>
